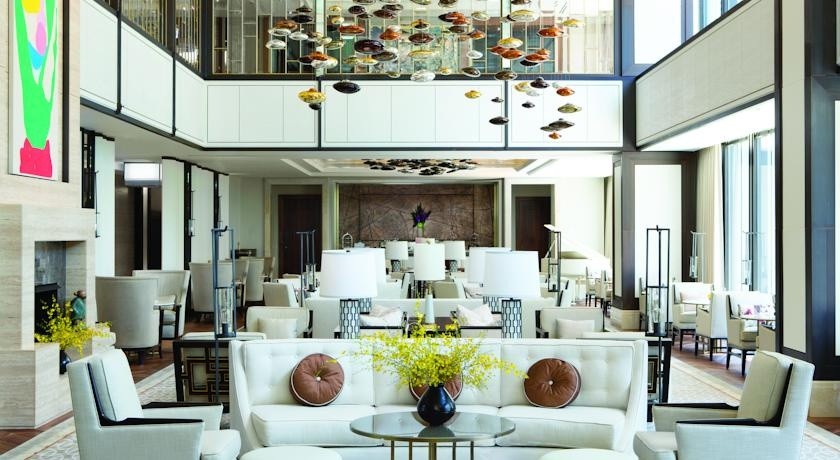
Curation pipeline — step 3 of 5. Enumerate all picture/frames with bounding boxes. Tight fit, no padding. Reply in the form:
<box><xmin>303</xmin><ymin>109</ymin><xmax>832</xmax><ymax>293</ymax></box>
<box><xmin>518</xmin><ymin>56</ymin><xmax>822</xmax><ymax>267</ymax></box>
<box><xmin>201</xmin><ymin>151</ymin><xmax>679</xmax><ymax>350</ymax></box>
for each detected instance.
<box><xmin>7</xmin><ymin>0</ymin><xmax>59</xmax><ymax>181</ymax></box>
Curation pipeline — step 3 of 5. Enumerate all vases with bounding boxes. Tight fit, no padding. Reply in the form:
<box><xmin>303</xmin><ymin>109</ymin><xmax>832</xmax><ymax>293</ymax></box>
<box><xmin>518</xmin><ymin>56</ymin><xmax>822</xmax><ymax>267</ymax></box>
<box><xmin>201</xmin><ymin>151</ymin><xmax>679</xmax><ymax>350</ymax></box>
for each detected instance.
<box><xmin>416</xmin><ymin>382</ymin><xmax>457</xmax><ymax>427</ymax></box>
<box><xmin>58</xmin><ymin>350</ymin><xmax>72</xmax><ymax>374</ymax></box>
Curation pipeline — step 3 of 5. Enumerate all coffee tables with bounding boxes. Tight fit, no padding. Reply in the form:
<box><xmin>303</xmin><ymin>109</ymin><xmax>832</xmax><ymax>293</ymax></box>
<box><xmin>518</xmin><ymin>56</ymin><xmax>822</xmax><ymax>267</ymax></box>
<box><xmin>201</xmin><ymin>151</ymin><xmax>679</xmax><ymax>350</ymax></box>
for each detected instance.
<box><xmin>349</xmin><ymin>411</ymin><xmax>517</xmax><ymax>460</ymax></box>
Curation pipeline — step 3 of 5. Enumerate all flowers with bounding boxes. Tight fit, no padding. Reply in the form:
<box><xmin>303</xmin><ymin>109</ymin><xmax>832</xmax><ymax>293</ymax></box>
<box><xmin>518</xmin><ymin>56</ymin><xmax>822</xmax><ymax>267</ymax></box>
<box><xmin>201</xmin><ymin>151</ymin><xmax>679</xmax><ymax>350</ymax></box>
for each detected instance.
<box><xmin>325</xmin><ymin>293</ymin><xmax>529</xmax><ymax>389</ymax></box>
<box><xmin>34</xmin><ymin>294</ymin><xmax>113</xmax><ymax>359</ymax></box>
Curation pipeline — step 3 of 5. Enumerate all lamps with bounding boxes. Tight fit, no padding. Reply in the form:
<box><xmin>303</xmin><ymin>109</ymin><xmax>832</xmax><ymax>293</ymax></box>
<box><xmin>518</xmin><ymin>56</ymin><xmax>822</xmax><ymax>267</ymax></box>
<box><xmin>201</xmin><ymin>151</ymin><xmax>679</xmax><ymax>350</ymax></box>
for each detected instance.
<box><xmin>263</xmin><ymin>0</ymin><xmax>584</xmax><ymax>140</ymax></box>
<box><xmin>646</xmin><ymin>286</ymin><xmax>668</xmax><ymax>337</ymax></box>
<box><xmin>319</xmin><ymin>236</ymin><xmax>539</xmax><ymax>340</ymax></box>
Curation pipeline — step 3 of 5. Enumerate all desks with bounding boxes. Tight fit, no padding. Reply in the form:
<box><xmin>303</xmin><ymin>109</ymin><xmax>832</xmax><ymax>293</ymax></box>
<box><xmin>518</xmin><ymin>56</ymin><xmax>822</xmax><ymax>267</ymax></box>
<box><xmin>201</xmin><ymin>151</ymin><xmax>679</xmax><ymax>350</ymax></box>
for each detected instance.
<box><xmin>153</xmin><ymin>305</ymin><xmax>173</xmax><ymax>348</ymax></box>
<box><xmin>740</xmin><ymin>314</ymin><xmax>776</xmax><ymax>337</ymax></box>
<box><xmin>682</xmin><ymin>299</ymin><xmax>718</xmax><ymax>355</ymax></box>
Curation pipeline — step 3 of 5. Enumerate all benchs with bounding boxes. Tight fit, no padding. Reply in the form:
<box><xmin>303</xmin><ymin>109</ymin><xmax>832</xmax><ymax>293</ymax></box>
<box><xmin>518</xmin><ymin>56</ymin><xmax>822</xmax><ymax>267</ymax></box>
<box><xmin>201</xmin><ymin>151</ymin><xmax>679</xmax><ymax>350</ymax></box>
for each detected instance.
<box><xmin>225</xmin><ymin>337</ymin><xmax>650</xmax><ymax>460</ymax></box>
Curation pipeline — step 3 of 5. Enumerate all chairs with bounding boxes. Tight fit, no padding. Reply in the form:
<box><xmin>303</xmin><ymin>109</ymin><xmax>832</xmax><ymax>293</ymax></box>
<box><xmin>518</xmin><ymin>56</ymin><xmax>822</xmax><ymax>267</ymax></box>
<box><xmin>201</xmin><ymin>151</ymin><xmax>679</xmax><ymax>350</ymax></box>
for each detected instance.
<box><xmin>576</xmin><ymin>337</ymin><xmax>675</xmax><ymax>423</ymax></box>
<box><xmin>758</xmin><ymin>323</ymin><xmax>776</xmax><ymax>353</ymax></box>
<box><xmin>671</xmin><ymin>281</ymin><xmax>715</xmax><ymax>351</ymax></box>
<box><xmin>722</xmin><ymin>293</ymin><xmax>776</xmax><ymax>377</ymax></box>
<box><xmin>132</xmin><ymin>270</ymin><xmax>191</xmax><ymax>340</ymax></box>
<box><xmin>173</xmin><ymin>331</ymin><xmax>268</xmax><ymax>430</ymax></box>
<box><xmin>189</xmin><ymin>256</ymin><xmax>613</xmax><ymax>340</ymax></box>
<box><xmin>633</xmin><ymin>348</ymin><xmax>815</xmax><ymax>460</ymax></box>
<box><xmin>66</xmin><ymin>347</ymin><xmax>243</xmax><ymax>460</ymax></box>
<box><xmin>637</xmin><ymin>275</ymin><xmax>676</xmax><ymax>332</ymax></box>
<box><xmin>92</xmin><ymin>276</ymin><xmax>159</xmax><ymax>365</ymax></box>
<box><xmin>694</xmin><ymin>290</ymin><xmax>753</xmax><ymax>361</ymax></box>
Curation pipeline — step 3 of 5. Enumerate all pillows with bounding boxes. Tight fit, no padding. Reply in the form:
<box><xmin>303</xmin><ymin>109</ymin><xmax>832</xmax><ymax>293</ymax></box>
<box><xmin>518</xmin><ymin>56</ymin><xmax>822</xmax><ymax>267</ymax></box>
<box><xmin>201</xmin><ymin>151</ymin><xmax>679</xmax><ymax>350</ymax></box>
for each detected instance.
<box><xmin>289</xmin><ymin>352</ymin><xmax>346</xmax><ymax>409</ymax></box>
<box><xmin>679</xmin><ymin>289</ymin><xmax>705</xmax><ymax>311</ymax></box>
<box><xmin>737</xmin><ymin>303</ymin><xmax>775</xmax><ymax>330</ymax></box>
<box><xmin>409</xmin><ymin>354</ymin><xmax>465</xmax><ymax>404</ymax></box>
<box><xmin>523</xmin><ymin>358</ymin><xmax>582</xmax><ymax>410</ymax></box>
